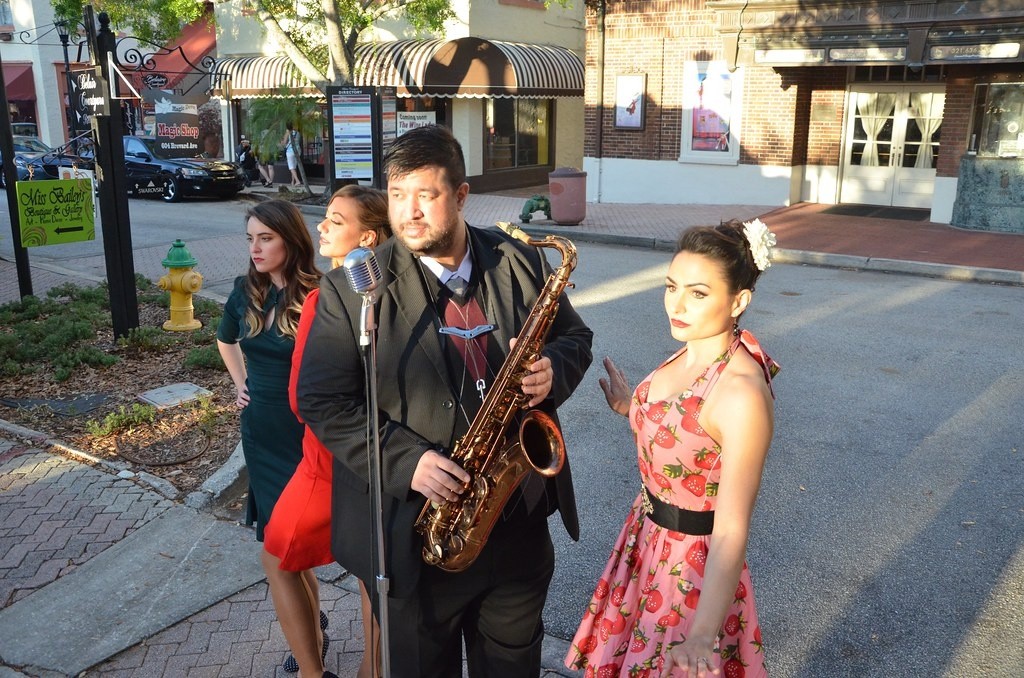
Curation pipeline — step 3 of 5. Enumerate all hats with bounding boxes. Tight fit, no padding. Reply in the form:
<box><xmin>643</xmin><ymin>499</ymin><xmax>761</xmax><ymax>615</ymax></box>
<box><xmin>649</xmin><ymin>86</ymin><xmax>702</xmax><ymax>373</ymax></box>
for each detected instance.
<box><xmin>241</xmin><ymin>139</ymin><xmax>249</xmax><ymax>143</ymax></box>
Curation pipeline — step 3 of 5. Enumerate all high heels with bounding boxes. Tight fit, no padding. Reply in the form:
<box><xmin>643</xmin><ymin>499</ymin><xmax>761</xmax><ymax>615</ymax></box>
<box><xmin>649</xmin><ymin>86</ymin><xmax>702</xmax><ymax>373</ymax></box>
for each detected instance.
<box><xmin>319</xmin><ymin>610</ymin><xmax>329</xmax><ymax>631</ymax></box>
<box><xmin>283</xmin><ymin>631</ymin><xmax>329</xmax><ymax>673</ymax></box>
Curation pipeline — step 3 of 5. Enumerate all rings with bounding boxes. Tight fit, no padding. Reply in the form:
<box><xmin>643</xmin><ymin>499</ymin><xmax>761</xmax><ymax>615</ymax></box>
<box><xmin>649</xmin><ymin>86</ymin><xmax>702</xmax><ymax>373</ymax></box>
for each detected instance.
<box><xmin>697</xmin><ymin>656</ymin><xmax>707</xmax><ymax>664</ymax></box>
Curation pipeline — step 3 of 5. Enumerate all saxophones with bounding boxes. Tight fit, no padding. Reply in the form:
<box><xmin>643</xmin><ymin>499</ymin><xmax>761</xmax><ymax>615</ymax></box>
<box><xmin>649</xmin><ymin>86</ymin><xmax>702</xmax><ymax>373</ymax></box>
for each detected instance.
<box><xmin>410</xmin><ymin>215</ymin><xmax>579</xmax><ymax>574</ymax></box>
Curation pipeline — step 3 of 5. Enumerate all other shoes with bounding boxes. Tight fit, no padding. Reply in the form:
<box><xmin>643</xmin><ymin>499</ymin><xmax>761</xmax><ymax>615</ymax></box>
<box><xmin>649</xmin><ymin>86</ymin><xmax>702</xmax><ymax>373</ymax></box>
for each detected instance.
<box><xmin>295</xmin><ymin>182</ymin><xmax>301</xmax><ymax>187</ymax></box>
<box><xmin>264</xmin><ymin>180</ymin><xmax>273</xmax><ymax>188</ymax></box>
<box><xmin>291</xmin><ymin>182</ymin><xmax>294</xmax><ymax>185</ymax></box>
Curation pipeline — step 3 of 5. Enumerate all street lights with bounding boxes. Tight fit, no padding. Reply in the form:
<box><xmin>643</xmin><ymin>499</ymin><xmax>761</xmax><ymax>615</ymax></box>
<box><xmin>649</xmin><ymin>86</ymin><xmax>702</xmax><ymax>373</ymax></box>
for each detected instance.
<box><xmin>52</xmin><ymin>16</ymin><xmax>78</xmax><ymax>155</ymax></box>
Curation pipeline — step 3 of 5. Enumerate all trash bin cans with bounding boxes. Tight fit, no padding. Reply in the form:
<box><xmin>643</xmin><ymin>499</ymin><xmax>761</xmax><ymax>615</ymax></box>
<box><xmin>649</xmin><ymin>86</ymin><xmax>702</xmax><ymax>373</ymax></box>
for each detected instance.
<box><xmin>547</xmin><ymin>168</ymin><xmax>588</xmax><ymax>225</ymax></box>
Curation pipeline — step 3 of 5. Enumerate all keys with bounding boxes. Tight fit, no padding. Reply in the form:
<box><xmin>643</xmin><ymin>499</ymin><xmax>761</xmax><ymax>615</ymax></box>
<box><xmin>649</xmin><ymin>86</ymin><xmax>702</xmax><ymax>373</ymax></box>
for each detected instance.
<box><xmin>476</xmin><ymin>378</ymin><xmax>486</xmax><ymax>402</ymax></box>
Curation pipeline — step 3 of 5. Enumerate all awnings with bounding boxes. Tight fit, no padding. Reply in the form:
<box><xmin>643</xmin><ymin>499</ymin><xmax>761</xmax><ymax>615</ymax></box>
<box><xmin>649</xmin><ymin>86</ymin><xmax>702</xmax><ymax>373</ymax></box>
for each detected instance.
<box><xmin>208</xmin><ymin>37</ymin><xmax>586</xmax><ymax>99</ymax></box>
<box><xmin>0</xmin><ymin>65</ymin><xmax>37</xmax><ymax>102</ymax></box>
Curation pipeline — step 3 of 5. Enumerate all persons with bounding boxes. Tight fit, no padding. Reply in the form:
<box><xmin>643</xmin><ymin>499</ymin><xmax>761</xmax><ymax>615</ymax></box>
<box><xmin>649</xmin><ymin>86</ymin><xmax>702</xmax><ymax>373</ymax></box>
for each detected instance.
<box><xmin>261</xmin><ymin>184</ymin><xmax>394</xmax><ymax>678</ymax></box>
<box><xmin>235</xmin><ymin>129</ymin><xmax>275</xmax><ymax>187</ymax></box>
<box><xmin>564</xmin><ymin>218</ymin><xmax>782</xmax><ymax>678</ymax></box>
<box><xmin>278</xmin><ymin>121</ymin><xmax>302</xmax><ymax>186</ymax></box>
<box><xmin>296</xmin><ymin>126</ymin><xmax>594</xmax><ymax>678</ymax></box>
<box><xmin>213</xmin><ymin>200</ymin><xmax>324</xmax><ymax>674</ymax></box>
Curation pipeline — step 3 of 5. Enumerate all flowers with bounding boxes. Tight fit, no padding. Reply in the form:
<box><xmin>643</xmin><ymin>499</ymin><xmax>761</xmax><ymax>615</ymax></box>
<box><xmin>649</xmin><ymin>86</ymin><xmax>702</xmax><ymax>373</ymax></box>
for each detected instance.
<box><xmin>197</xmin><ymin>99</ymin><xmax>222</xmax><ymax>137</ymax></box>
<box><xmin>743</xmin><ymin>218</ymin><xmax>777</xmax><ymax>270</ymax></box>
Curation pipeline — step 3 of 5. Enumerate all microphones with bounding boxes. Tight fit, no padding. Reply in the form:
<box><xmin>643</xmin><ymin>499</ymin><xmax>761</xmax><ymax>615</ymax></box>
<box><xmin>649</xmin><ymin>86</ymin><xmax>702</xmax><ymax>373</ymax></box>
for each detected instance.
<box><xmin>342</xmin><ymin>247</ymin><xmax>384</xmax><ymax>357</ymax></box>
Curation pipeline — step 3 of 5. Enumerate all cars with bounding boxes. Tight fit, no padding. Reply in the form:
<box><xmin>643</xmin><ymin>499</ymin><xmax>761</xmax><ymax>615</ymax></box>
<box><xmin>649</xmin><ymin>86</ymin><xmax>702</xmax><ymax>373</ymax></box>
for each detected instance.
<box><xmin>80</xmin><ymin>135</ymin><xmax>247</xmax><ymax>202</ymax></box>
<box><xmin>0</xmin><ymin>135</ymin><xmax>84</xmax><ymax>189</ymax></box>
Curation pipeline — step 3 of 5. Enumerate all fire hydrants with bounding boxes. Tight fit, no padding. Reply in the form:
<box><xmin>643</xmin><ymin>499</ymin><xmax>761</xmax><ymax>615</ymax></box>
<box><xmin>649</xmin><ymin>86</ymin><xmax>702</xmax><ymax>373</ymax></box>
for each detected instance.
<box><xmin>157</xmin><ymin>238</ymin><xmax>204</xmax><ymax>331</ymax></box>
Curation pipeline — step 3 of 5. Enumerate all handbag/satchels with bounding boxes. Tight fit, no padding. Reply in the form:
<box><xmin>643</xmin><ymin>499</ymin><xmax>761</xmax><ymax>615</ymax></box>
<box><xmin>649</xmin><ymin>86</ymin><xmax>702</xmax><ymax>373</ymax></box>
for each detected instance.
<box><xmin>281</xmin><ymin>147</ymin><xmax>287</xmax><ymax>161</ymax></box>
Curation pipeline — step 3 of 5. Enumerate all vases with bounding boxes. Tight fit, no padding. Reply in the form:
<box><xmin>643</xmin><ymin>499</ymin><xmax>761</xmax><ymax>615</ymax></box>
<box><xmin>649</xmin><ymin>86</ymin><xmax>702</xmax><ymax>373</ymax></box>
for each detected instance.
<box><xmin>205</xmin><ymin>134</ymin><xmax>220</xmax><ymax>158</ymax></box>
